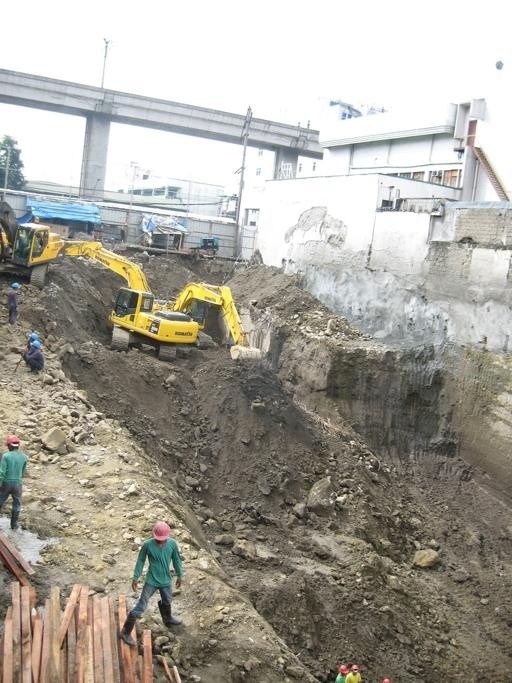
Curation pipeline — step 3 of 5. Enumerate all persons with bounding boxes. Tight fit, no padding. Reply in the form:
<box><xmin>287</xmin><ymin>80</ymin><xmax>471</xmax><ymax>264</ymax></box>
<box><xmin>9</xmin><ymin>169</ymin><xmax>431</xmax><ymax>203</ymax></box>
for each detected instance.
<box><xmin>34</xmin><ymin>216</ymin><xmax>40</xmax><ymax>223</ymax></box>
<box><xmin>25</xmin><ymin>340</ymin><xmax>45</xmax><ymax>374</ymax></box>
<box><xmin>345</xmin><ymin>663</ymin><xmax>362</xmax><ymax>683</ymax></box>
<box><xmin>334</xmin><ymin>664</ymin><xmax>349</xmax><ymax>683</ymax></box>
<box><xmin>120</xmin><ymin>223</ymin><xmax>129</xmax><ymax>243</ymax></box>
<box><xmin>8</xmin><ymin>282</ymin><xmax>20</xmax><ymax>325</ymax></box>
<box><xmin>118</xmin><ymin>522</ymin><xmax>184</xmax><ymax>646</ymax></box>
<box><xmin>21</xmin><ymin>332</ymin><xmax>40</xmax><ymax>364</ymax></box>
<box><xmin>1</xmin><ymin>435</ymin><xmax>28</xmax><ymax>528</ymax></box>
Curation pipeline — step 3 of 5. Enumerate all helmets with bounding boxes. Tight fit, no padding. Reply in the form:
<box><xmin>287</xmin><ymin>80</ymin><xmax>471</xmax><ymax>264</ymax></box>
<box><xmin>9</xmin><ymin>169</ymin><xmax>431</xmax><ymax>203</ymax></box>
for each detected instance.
<box><xmin>30</xmin><ymin>340</ymin><xmax>43</xmax><ymax>350</ymax></box>
<box><xmin>11</xmin><ymin>282</ymin><xmax>21</xmax><ymax>288</ymax></box>
<box><xmin>28</xmin><ymin>332</ymin><xmax>39</xmax><ymax>339</ymax></box>
<box><xmin>5</xmin><ymin>434</ymin><xmax>21</xmax><ymax>445</ymax></box>
<box><xmin>152</xmin><ymin>521</ymin><xmax>171</xmax><ymax>541</ymax></box>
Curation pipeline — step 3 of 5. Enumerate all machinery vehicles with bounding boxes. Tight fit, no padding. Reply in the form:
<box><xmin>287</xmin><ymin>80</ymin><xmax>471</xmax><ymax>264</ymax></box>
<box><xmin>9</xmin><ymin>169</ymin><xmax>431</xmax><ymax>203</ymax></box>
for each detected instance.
<box><xmin>108</xmin><ymin>282</ymin><xmax>262</xmax><ymax>362</ymax></box>
<box><xmin>0</xmin><ymin>222</ymin><xmax>152</xmax><ymax>293</ymax></box>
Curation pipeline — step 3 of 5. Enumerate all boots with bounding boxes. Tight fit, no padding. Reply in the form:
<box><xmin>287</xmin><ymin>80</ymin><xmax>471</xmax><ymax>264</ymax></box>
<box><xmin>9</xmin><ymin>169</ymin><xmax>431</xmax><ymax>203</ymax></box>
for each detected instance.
<box><xmin>157</xmin><ymin>600</ymin><xmax>183</xmax><ymax>625</ymax></box>
<box><xmin>11</xmin><ymin>510</ymin><xmax>21</xmax><ymax>529</ymax></box>
<box><xmin>119</xmin><ymin>613</ymin><xmax>138</xmax><ymax>646</ymax></box>
